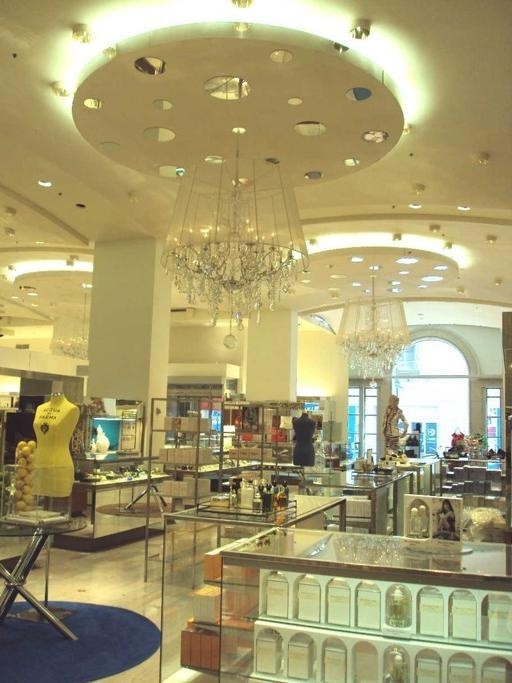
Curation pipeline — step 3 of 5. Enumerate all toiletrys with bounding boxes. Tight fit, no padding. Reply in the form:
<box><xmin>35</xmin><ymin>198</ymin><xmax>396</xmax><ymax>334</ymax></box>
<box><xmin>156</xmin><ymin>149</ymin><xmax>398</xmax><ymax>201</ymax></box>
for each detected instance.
<box><xmin>409</xmin><ymin>505</ymin><xmax>428</xmax><ymax>536</ymax></box>
<box><xmin>230</xmin><ymin>475</ymin><xmax>289</xmax><ymax>514</ymax></box>
<box><xmin>230</xmin><ymin>477</ymin><xmax>288</xmax><ymax>509</ymax></box>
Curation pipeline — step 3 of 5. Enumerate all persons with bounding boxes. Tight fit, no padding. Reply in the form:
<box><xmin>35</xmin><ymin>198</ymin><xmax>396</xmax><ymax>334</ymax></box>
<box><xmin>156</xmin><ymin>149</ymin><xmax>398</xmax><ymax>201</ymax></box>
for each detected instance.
<box><xmin>382</xmin><ymin>395</ymin><xmax>409</xmax><ymax>451</ymax></box>
<box><xmin>435</xmin><ymin>499</ymin><xmax>455</xmax><ymax>533</ymax></box>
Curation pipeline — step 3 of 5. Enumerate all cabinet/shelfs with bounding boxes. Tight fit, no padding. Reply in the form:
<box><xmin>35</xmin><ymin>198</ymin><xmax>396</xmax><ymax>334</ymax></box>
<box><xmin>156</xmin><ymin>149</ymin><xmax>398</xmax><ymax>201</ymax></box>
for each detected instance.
<box><xmin>436</xmin><ymin>454</ymin><xmax>510</xmax><ymax>501</ymax></box>
<box><xmin>166</xmin><ymin>458</ymin><xmax>435</xmax><ymax>584</ymax></box>
<box><xmin>181</xmin><ymin>526</ymin><xmax>511</xmax><ymax>681</ymax></box>
<box><xmin>146</xmin><ymin>399</ymin><xmax>347</xmax><ymax>513</ymax></box>
<box><xmin>45</xmin><ymin>453</ymin><xmax>171</xmax><ymax>553</ymax></box>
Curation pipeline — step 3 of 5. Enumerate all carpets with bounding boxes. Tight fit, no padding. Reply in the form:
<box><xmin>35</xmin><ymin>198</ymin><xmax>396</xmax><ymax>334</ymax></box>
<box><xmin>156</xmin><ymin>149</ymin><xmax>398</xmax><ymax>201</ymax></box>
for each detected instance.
<box><xmin>0</xmin><ymin>599</ymin><xmax>162</xmax><ymax>682</ymax></box>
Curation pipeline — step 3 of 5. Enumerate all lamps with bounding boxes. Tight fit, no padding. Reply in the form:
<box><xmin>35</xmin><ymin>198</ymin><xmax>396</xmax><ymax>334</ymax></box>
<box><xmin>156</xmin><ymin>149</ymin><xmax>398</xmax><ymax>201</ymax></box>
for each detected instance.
<box><xmin>334</xmin><ymin>272</ymin><xmax>414</xmax><ymax>389</ymax></box>
<box><xmin>158</xmin><ymin>128</ymin><xmax>312</xmax><ymax>350</ymax></box>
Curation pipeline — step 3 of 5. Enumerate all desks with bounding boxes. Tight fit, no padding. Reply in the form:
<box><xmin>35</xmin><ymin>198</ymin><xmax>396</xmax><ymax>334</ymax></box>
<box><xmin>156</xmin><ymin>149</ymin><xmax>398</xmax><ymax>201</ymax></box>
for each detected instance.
<box><xmin>0</xmin><ymin>512</ymin><xmax>88</xmax><ymax>639</ymax></box>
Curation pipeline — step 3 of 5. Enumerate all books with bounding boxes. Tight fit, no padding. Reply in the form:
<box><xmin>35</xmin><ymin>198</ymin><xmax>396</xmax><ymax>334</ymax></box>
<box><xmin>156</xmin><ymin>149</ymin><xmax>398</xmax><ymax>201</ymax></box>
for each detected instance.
<box><xmin>433</xmin><ymin>497</ymin><xmax>463</xmax><ymax>542</ymax></box>
